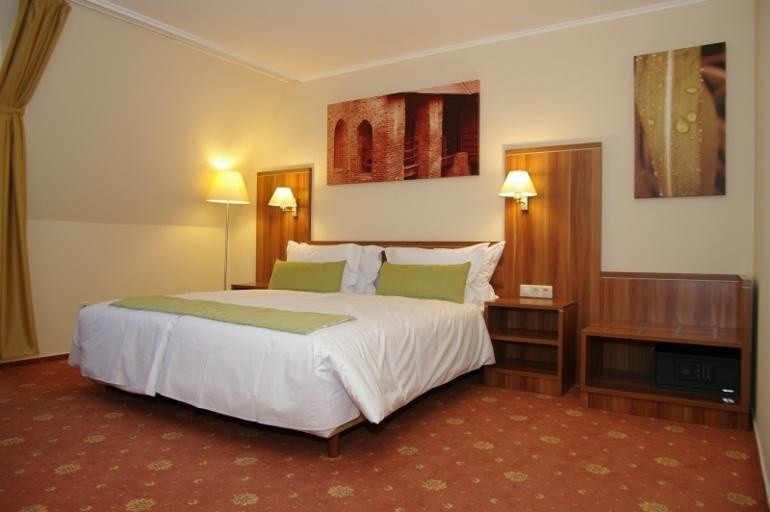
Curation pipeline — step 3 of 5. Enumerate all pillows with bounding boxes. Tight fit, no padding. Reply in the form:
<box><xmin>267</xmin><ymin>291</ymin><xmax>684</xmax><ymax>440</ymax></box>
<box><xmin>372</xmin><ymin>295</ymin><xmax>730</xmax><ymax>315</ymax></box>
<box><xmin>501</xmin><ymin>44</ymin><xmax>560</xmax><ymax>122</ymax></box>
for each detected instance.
<box><xmin>473</xmin><ymin>241</ymin><xmax>506</xmax><ymax>302</ymax></box>
<box><xmin>285</xmin><ymin>240</ymin><xmax>361</xmax><ymax>292</ymax></box>
<box><xmin>377</xmin><ymin>261</ymin><xmax>471</xmax><ymax>304</ymax></box>
<box><xmin>268</xmin><ymin>258</ymin><xmax>347</xmax><ymax>292</ymax></box>
<box><xmin>300</xmin><ymin>242</ymin><xmax>385</xmax><ymax>295</ymax></box>
<box><xmin>386</xmin><ymin>243</ymin><xmax>487</xmax><ymax>304</ymax></box>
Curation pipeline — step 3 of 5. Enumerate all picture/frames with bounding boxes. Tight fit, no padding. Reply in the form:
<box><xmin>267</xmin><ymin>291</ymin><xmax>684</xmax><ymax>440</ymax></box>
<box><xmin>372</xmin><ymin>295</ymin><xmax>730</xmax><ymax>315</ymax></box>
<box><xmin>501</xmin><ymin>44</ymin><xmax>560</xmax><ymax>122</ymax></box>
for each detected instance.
<box><xmin>327</xmin><ymin>80</ymin><xmax>480</xmax><ymax>186</ymax></box>
<box><xmin>633</xmin><ymin>41</ymin><xmax>727</xmax><ymax>199</ymax></box>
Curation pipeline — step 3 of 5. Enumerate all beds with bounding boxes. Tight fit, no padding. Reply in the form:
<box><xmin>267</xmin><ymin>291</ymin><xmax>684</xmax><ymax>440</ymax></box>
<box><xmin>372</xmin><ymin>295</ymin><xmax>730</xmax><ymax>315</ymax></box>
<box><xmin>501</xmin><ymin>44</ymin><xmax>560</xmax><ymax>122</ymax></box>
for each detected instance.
<box><xmin>68</xmin><ymin>241</ymin><xmax>500</xmax><ymax>458</ymax></box>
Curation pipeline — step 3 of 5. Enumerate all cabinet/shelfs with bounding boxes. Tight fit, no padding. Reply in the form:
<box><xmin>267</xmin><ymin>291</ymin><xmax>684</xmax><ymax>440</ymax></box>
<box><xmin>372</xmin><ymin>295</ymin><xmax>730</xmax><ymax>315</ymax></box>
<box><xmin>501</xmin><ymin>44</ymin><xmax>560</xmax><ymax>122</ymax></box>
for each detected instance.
<box><xmin>577</xmin><ymin>272</ymin><xmax>751</xmax><ymax>432</ymax></box>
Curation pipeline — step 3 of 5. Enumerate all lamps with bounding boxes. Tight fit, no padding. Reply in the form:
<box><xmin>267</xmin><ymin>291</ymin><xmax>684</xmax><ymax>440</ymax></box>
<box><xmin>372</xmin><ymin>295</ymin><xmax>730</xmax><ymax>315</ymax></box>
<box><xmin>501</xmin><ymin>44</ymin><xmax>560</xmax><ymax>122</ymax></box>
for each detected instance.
<box><xmin>206</xmin><ymin>171</ymin><xmax>249</xmax><ymax>290</ymax></box>
<box><xmin>267</xmin><ymin>187</ymin><xmax>297</xmax><ymax>217</ymax></box>
<box><xmin>499</xmin><ymin>171</ymin><xmax>537</xmax><ymax>212</ymax></box>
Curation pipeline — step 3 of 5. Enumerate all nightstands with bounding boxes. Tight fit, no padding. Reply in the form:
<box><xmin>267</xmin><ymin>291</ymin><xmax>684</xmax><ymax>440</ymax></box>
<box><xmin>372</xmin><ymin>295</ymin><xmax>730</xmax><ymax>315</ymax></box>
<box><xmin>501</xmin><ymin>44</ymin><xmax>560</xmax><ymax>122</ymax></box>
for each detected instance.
<box><xmin>231</xmin><ymin>282</ymin><xmax>268</xmax><ymax>289</ymax></box>
<box><xmin>482</xmin><ymin>297</ymin><xmax>578</xmax><ymax>396</ymax></box>
